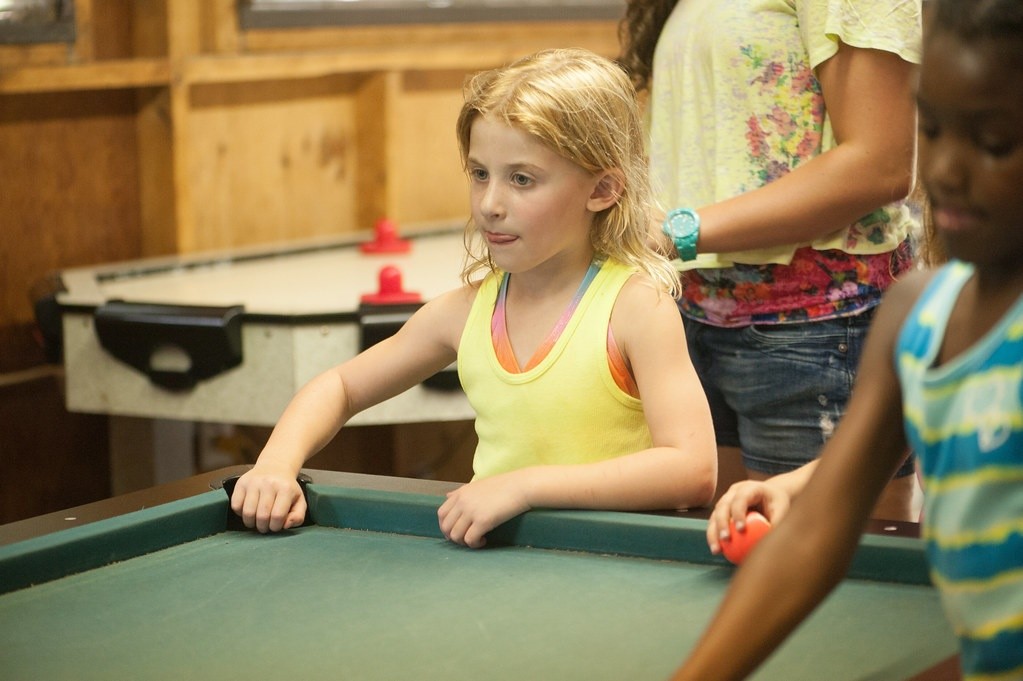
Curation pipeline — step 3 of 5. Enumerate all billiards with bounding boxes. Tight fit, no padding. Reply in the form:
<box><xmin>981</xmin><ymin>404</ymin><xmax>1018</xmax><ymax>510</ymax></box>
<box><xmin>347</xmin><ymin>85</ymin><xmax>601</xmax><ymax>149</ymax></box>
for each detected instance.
<box><xmin>719</xmin><ymin>510</ymin><xmax>772</xmax><ymax>566</ymax></box>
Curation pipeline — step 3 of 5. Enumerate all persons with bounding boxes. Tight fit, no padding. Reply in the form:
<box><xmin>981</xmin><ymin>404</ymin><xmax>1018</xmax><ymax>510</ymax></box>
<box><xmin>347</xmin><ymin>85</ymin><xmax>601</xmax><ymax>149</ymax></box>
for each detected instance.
<box><xmin>229</xmin><ymin>47</ymin><xmax>719</xmax><ymax>553</ymax></box>
<box><xmin>667</xmin><ymin>0</ymin><xmax>1023</xmax><ymax>681</ymax></box>
<box><xmin>609</xmin><ymin>0</ymin><xmax>933</xmax><ymax>533</ymax></box>
<box><xmin>708</xmin><ymin>457</ymin><xmax>821</xmax><ymax>550</ymax></box>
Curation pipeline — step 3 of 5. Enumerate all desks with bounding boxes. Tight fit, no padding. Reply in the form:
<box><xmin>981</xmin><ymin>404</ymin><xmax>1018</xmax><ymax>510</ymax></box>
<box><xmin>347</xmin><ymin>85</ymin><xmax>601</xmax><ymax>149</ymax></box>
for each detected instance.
<box><xmin>35</xmin><ymin>216</ymin><xmax>474</xmax><ymax>499</ymax></box>
<box><xmin>0</xmin><ymin>463</ymin><xmax>962</xmax><ymax>680</ymax></box>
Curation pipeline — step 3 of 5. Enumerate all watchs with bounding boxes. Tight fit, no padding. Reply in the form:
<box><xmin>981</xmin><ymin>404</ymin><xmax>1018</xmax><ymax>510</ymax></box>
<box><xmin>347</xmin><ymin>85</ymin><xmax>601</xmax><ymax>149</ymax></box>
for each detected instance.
<box><xmin>663</xmin><ymin>209</ymin><xmax>700</xmax><ymax>262</ymax></box>
